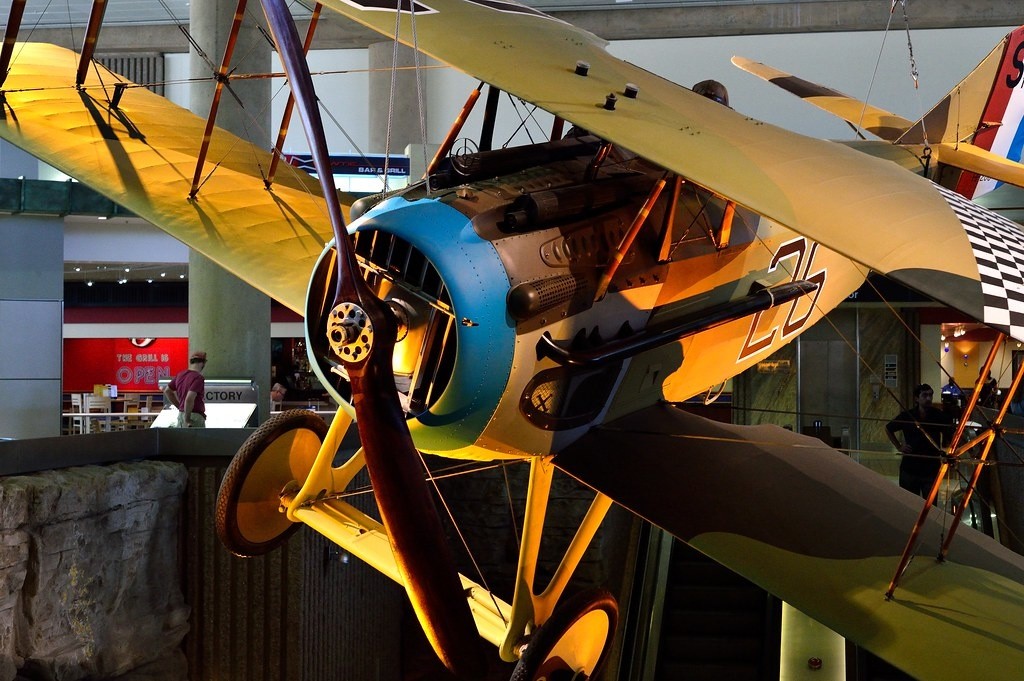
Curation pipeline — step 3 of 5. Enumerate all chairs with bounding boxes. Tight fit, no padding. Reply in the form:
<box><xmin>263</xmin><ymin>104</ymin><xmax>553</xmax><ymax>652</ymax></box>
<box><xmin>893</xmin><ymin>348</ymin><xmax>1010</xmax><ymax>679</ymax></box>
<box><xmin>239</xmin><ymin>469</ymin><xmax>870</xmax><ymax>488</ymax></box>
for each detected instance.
<box><xmin>65</xmin><ymin>389</ymin><xmax>164</xmax><ymax>433</ymax></box>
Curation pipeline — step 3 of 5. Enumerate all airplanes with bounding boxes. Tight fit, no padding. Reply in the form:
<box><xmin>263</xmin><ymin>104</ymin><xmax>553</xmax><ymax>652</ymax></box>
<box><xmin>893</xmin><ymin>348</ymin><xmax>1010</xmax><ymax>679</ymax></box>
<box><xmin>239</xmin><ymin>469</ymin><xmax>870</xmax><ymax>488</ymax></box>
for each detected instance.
<box><xmin>0</xmin><ymin>0</ymin><xmax>1024</xmax><ymax>680</ymax></box>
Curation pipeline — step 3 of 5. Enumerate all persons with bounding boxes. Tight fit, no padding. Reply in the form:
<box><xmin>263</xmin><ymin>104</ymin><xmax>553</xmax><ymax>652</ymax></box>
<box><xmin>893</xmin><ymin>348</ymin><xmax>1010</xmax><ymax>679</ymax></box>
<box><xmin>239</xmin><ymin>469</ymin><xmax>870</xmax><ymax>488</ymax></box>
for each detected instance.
<box><xmin>975</xmin><ymin>367</ymin><xmax>998</xmax><ymax>408</ymax></box>
<box><xmin>164</xmin><ymin>352</ymin><xmax>207</xmax><ymax>428</ymax></box>
<box><xmin>942</xmin><ymin>377</ymin><xmax>960</xmax><ymax>395</ymax></box>
<box><xmin>886</xmin><ymin>383</ymin><xmax>953</xmax><ymax>505</ymax></box>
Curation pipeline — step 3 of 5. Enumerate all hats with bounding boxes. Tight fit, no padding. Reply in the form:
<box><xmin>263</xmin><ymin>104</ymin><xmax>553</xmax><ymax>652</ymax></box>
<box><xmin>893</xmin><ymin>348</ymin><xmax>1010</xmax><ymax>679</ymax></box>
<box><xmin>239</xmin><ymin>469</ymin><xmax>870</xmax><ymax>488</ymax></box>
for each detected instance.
<box><xmin>190</xmin><ymin>352</ymin><xmax>206</xmax><ymax>359</ymax></box>
